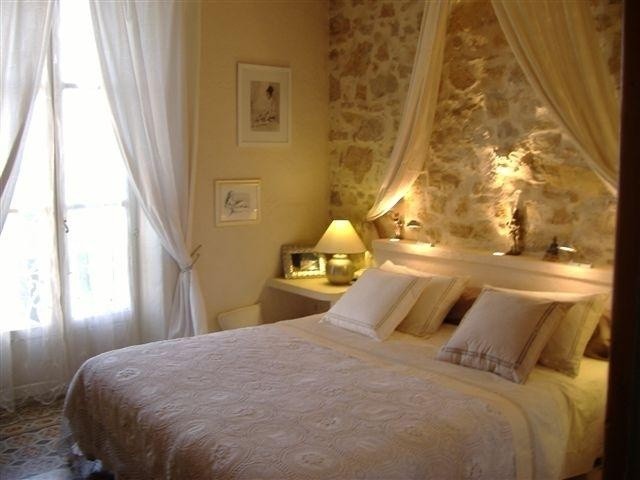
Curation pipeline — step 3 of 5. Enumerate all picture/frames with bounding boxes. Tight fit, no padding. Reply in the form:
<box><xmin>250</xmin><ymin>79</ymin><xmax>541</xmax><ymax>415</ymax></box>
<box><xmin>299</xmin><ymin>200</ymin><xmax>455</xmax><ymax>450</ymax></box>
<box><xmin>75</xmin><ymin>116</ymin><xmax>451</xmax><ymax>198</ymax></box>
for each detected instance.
<box><xmin>282</xmin><ymin>245</ymin><xmax>327</xmax><ymax>279</ymax></box>
<box><xmin>237</xmin><ymin>61</ymin><xmax>292</xmax><ymax>149</ymax></box>
<box><xmin>214</xmin><ymin>179</ymin><xmax>262</xmax><ymax>227</ymax></box>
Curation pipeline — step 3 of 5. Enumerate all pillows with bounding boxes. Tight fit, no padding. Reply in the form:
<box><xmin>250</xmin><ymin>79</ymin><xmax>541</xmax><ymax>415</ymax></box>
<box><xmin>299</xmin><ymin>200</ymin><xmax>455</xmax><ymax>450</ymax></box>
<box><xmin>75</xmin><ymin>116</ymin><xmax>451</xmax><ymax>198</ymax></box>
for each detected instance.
<box><xmin>322</xmin><ymin>259</ymin><xmax>613</xmax><ymax>386</ymax></box>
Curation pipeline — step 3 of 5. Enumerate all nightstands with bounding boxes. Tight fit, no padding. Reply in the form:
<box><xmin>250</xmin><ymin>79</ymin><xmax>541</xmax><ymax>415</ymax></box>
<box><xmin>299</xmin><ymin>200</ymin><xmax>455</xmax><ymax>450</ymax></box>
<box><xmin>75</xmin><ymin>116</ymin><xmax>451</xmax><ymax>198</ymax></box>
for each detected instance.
<box><xmin>267</xmin><ymin>276</ymin><xmax>356</xmax><ymax>309</ymax></box>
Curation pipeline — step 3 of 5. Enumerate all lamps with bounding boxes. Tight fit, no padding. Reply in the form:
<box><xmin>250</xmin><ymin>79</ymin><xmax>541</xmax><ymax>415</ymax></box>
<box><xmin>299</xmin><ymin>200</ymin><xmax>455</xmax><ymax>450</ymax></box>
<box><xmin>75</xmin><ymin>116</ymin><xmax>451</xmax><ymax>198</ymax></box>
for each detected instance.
<box><xmin>557</xmin><ymin>240</ymin><xmax>590</xmax><ymax>270</ymax></box>
<box><xmin>312</xmin><ymin>220</ymin><xmax>367</xmax><ymax>285</ymax></box>
<box><xmin>406</xmin><ymin>220</ymin><xmax>435</xmax><ymax>248</ymax></box>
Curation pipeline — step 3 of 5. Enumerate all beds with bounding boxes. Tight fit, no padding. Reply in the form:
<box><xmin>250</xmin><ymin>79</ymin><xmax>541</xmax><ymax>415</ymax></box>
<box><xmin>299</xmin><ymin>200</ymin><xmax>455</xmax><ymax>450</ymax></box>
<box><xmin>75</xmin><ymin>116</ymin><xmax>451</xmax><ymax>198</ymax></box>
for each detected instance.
<box><xmin>62</xmin><ymin>237</ymin><xmax>614</xmax><ymax>480</ymax></box>
